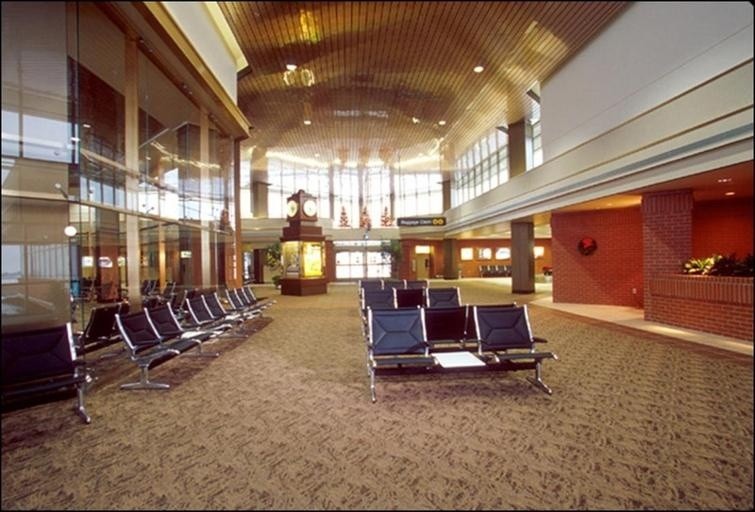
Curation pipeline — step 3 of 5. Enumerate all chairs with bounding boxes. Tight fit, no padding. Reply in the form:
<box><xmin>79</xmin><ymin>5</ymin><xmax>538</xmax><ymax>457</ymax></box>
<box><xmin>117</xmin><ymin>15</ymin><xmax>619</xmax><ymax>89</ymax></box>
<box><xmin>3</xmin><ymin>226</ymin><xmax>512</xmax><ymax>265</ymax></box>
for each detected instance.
<box><xmin>241</xmin><ymin>284</ymin><xmax>278</xmax><ymax>307</ymax></box>
<box><xmin>366</xmin><ymin>307</ymin><xmax>439</xmax><ymax>403</ymax></box>
<box><xmin>200</xmin><ymin>291</ymin><xmax>258</xmax><ymax>333</ymax></box>
<box><xmin>183</xmin><ymin>288</ymin><xmax>199</xmax><ymax>314</ymax></box>
<box><xmin>194</xmin><ymin>284</ymin><xmax>219</xmax><ymax>296</ymax></box>
<box><xmin>396</xmin><ymin>286</ymin><xmax>427</xmax><ymax>309</ymax></box>
<box><xmin>495</xmin><ymin>263</ymin><xmax>507</xmax><ymax>274</ymax></box>
<box><xmin>487</xmin><ymin>263</ymin><xmax>499</xmax><ymax>275</ymax></box>
<box><xmin>357</xmin><ymin>278</ymin><xmax>383</xmax><ymax>296</ymax></box>
<box><xmin>115</xmin><ymin>307</ymin><xmax>180</xmax><ymax>390</ymax></box>
<box><xmin>459</xmin><ymin>301</ymin><xmax>517</xmax><ymax>350</ymax></box>
<box><xmin>142</xmin><ymin>279</ymin><xmax>151</xmax><ymax>295</ymax></box>
<box><xmin>2</xmin><ymin>320</ymin><xmax>91</xmax><ymax>424</ymax></box>
<box><xmin>425</xmin><ymin>285</ymin><xmax>462</xmax><ymax>309</ymax></box>
<box><xmin>98</xmin><ymin>300</ymin><xmax>132</xmax><ymax>360</ymax></box>
<box><xmin>233</xmin><ymin>287</ymin><xmax>266</xmax><ymax>315</ymax></box>
<box><xmin>503</xmin><ymin>264</ymin><xmax>512</xmax><ymax>276</ymax></box>
<box><xmin>185</xmin><ymin>293</ymin><xmax>248</xmax><ymax>338</ymax></box>
<box><xmin>225</xmin><ymin>287</ymin><xmax>274</xmax><ymax>321</ymax></box>
<box><xmin>417</xmin><ymin>303</ymin><xmax>470</xmax><ymax>350</ymax></box>
<box><xmin>169</xmin><ymin>289</ymin><xmax>196</xmax><ymax>327</ymax></box>
<box><xmin>143</xmin><ymin>301</ymin><xmax>220</xmax><ymax>358</ymax></box>
<box><xmin>163</xmin><ymin>281</ymin><xmax>175</xmax><ymax>299</ymax></box>
<box><xmin>358</xmin><ymin>287</ymin><xmax>399</xmax><ymax>341</ymax></box>
<box><xmin>366</xmin><ymin>304</ymin><xmax>421</xmax><ymax>376</ymax></box>
<box><xmin>141</xmin><ymin>294</ymin><xmax>160</xmax><ymax>311</ymax></box>
<box><xmin>384</xmin><ymin>279</ymin><xmax>405</xmax><ymax>290</ymax></box>
<box><xmin>149</xmin><ymin>279</ymin><xmax>162</xmax><ymax>294</ymax></box>
<box><xmin>405</xmin><ymin>279</ymin><xmax>430</xmax><ymax>289</ymax></box>
<box><xmin>472</xmin><ymin>303</ymin><xmax>559</xmax><ymax>395</ymax></box>
<box><xmin>478</xmin><ymin>263</ymin><xmax>490</xmax><ymax>278</ymax></box>
<box><xmin>72</xmin><ymin>302</ymin><xmax>123</xmax><ymax>357</ymax></box>
